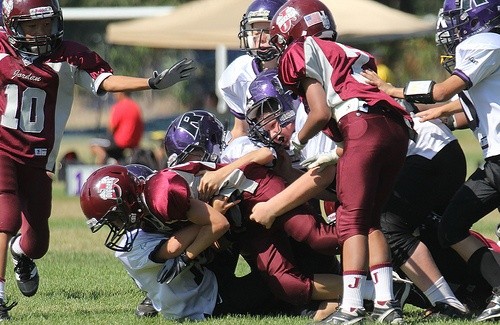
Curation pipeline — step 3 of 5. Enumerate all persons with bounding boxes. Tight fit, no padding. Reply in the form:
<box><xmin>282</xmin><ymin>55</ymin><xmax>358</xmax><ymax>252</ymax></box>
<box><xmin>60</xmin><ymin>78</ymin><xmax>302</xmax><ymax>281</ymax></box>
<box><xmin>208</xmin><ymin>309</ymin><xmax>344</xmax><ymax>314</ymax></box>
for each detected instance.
<box><xmin>80</xmin><ymin>0</ymin><xmax>500</xmax><ymax>325</ymax></box>
<box><xmin>0</xmin><ymin>0</ymin><xmax>197</xmax><ymax>322</ymax></box>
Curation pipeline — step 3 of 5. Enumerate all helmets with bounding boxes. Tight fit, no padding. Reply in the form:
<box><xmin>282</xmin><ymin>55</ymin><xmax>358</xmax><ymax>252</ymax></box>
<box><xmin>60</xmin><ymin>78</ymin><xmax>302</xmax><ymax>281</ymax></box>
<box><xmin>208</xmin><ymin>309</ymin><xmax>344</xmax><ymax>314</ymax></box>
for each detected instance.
<box><xmin>124</xmin><ymin>164</ymin><xmax>154</xmax><ymax>183</ymax></box>
<box><xmin>80</xmin><ymin>165</ymin><xmax>145</xmax><ymax>252</ymax></box>
<box><xmin>164</xmin><ymin>110</ymin><xmax>227</xmax><ymax>168</ymax></box>
<box><xmin>245</xmin><ymin>69</ymin><xmax>301</xmax><ymax>148</ymax></box>
<box><xmin>238</xmin><ymin>0</ymin><xmax>287</xmax><ymax>61</ymax></box>
<box><xmin>435</xmin><ymin>0</ymin><xmax>500</xmax><ymax>55</ymax></box>
<box><xmin>1</xmin><ymin>0</ymin><xmax>64</xmax><ymax>57</ymax></box>
<box><xmin>268</xmin><ymin>0</ymin><xmax>337</xmax><ymax>60</ymax></box>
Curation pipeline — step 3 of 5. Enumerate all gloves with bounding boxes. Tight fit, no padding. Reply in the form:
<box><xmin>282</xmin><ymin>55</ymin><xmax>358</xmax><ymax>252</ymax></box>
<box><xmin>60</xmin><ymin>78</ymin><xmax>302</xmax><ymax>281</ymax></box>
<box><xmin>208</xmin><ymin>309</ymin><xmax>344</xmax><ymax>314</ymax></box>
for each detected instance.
<box><xmin>156</xmin><ymin>251</ymin><xmax>194</xmax><ymax>284</ymax></box>
<box><xmin>299</xmin><ymin>148</ymin><xmax>339</xmax><ymax>172</ymax></box>
<box><xmin>148</xmin><ymin>58</ymin><xmax>197</xmax><ymax>89</ymax></box>
<box><xmin>290</xmin><ymin>132</ymin><xmax>307</xmax><ymax>152</ymax></box>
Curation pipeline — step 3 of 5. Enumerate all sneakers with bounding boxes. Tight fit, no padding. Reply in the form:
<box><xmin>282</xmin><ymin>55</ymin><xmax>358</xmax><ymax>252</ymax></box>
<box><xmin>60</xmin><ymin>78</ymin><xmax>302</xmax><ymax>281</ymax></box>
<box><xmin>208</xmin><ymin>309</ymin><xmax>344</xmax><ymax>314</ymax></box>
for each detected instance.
<box><xmin>9</xmin><ymin>233</ymin><xmax>39</xmax><ymax>297</ymax></box>
<box><xmin>321</xmin><ymin>307</ymin><xmax>368</xmax><ymax>325</ymax></box>
<box><xmin>477</xmin><ymin>286</ymin><xmax>500</xmax><ymax>322</ymax></box>
<box><xmin>391</xmin><ymin>271</ymin><xmax>431</xmax><ymax>308</ymax></box>
<box><xmin>0</xmin><ymin>297</ymin><xmax>10</xmax><ymax>320</ymax></box>
<box><xmin>137</xmin><ymin>296</ymin><xmax>158</xmax><ymax>317</ymax></box>
<box><xmin>369</xmin><ymin>300</ymin><xmax>404</xmax><ymax>325</ymax></box>
<box><xmin>424</xmin><ymin>301</ymin><xmax>471</xmax><ymax>321</ymax></box>
